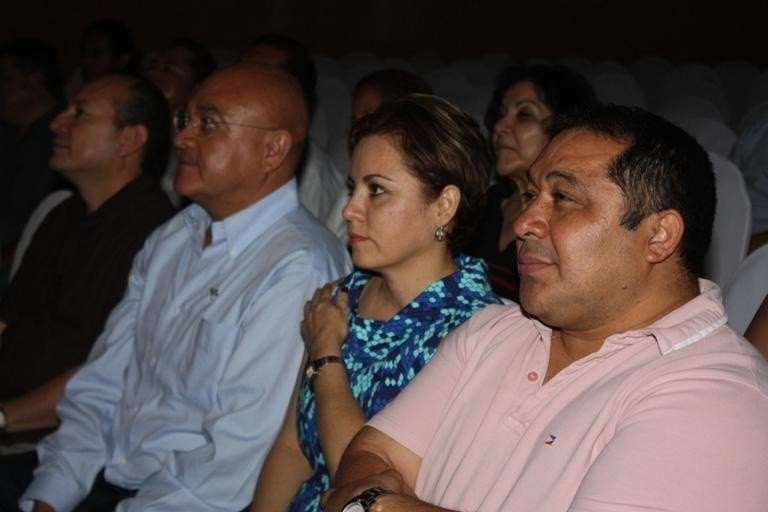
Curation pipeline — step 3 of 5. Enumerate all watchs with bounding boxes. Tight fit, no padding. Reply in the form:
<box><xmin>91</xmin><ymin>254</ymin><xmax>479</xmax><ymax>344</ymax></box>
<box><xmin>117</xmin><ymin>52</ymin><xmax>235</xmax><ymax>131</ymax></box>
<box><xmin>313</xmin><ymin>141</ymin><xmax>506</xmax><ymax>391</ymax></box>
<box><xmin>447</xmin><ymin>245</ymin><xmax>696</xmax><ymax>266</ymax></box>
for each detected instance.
<box><xmin>305</xmin><ymin>356</ymin><xmax>346</xmax><ymax>393</ymax></box>
<box><xmin>342</xmin><ymin>488</ymin><xmax>392</xmax><ymax>510</ymax></box>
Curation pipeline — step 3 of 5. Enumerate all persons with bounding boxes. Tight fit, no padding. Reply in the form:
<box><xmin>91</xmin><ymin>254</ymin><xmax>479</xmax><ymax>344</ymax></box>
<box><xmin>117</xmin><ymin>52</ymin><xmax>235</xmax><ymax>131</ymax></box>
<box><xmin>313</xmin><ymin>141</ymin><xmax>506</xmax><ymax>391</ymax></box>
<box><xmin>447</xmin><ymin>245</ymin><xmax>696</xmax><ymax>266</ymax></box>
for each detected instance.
<box><xmin>242</xmin><ymin>95</ymin><xmax>506</xmax><ymax>511</ymax></box>
<box><xmin>446</xmin><ymin>66</ymin><xmax>597</xmax><ymax>299</ymax></box>
<box><xmin>20</xmin><ymin>67</ymin><xmax>354</xmax><ymax>509</ymax></box>
<box><xmin>1</xmin><ymin>72</ymin><xmax>178</xmax><ymax>511</ymax></box>
<box><xmin>319</xmin><ymin>105</ymin><xmax>765</xmax><ymax>510</ymax></box>
<box><xmin>238</xmin><ymin>34</ymin><xmax>345</xmax><ymax>226</ymax></box>
<box><xmin>327</xmin><ymin>69</ymin><xmax>430</xmax><ymax>247</ymax></box>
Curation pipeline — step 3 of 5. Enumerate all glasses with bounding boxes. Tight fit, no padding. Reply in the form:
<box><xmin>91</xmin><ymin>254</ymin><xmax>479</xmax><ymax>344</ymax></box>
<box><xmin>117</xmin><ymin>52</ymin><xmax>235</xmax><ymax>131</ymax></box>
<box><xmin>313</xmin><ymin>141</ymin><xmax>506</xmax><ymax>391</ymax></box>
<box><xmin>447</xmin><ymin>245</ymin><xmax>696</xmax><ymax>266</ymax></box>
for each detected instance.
<box><xmin>171</xmin><ymin>111</ymin><xmax>280</xmax><ymax>138</ymax></box>
